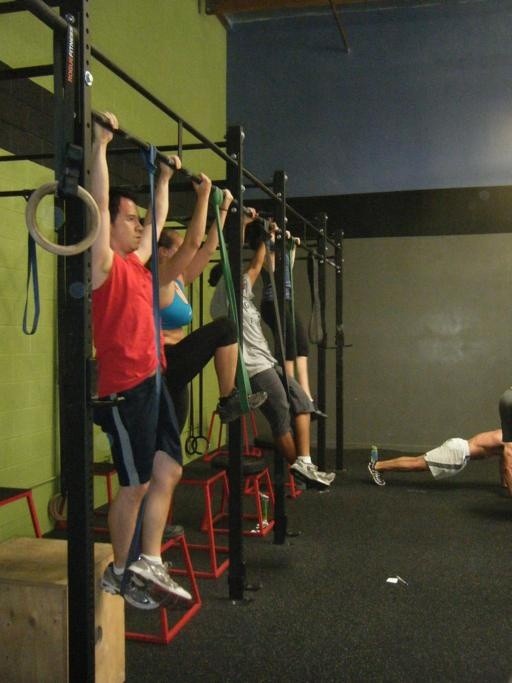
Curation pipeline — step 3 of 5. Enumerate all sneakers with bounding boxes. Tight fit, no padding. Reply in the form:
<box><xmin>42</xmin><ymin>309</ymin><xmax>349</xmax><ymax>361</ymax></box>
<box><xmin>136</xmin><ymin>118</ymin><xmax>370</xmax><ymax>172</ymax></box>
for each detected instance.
<box><xmin>162</xmin><ymin>524</ymin><xmax>184</xmax><ymax>538</ymax></box>
<box><xmin>216</xmin><ymin>390</ymin><xmax>268</xmax><ymax>424</ymax></box>
<box><xmin>312</xmin><ymin>400</ymin><xmax>329</xmax><ymax>418</ymax></box>
<box><xmin>100</xmin><ymin>553</ymin><xmax>193</xmax><ymax>610</ymax></box>
<box><xmin>367</xmin><ymin>460</ymin><xmax>386</xmax><ymax>486</ymax></box>
<box><xmin>289</xmin><ymin>458</ymin><xmax>336</xmax><ymax>489</ymax></box>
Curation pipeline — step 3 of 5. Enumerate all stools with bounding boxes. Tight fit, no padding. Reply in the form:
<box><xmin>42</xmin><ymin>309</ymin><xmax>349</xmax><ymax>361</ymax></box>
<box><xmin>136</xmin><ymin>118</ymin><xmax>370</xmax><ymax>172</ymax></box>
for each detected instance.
<box><xmin>201</xmin><ymin>455</ymin><xmax>275</xmax><ymax>538</ymax></box>
<box><xmin>0</xmin><ymin>486</ymin><xmax>43</xmax><ymax>538</ymax></box>
<box><xmin>166</xmin><ymin>466</ymin><xmax>232</xmax><ymax>579</ymax></box>
<box><xmin>57</xmin><ymin>462</ymin><xmax>120</xmax><ymax>533</ymax></box>
<box><xmin>124</xmin><ymin>526</ymin><xmax>202</xmax><ymax>645</ymax></box>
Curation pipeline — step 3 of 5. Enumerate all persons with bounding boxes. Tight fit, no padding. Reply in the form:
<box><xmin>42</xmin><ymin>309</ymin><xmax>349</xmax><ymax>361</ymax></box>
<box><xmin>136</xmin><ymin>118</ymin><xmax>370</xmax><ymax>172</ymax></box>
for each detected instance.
<box><xmin>367</xmin><ymin>429</ymin><xmax>512</xmax><ymax>498</ymax></box>
<box><xmin>210</xmin><ymin>207</ymin><xmax>336</xmax><ymax>488</ymax></box>
<box><xmin>90</xmin><ymin>110</ymin><xmax>196</xmax><ymax>612</ymax></box>
<box><xmin>158</xmin><ymin>172</ymin><xmax>268</xmax><ymax>541</ymax></box>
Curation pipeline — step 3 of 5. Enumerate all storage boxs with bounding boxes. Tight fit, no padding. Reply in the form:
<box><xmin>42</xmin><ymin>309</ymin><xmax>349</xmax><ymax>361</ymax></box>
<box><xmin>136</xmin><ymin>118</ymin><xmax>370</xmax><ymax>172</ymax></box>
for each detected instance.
<box><xmin>0</xmin><ymin>536</ymin><xmax>126</xmax><ymax>683</ymax></box>
